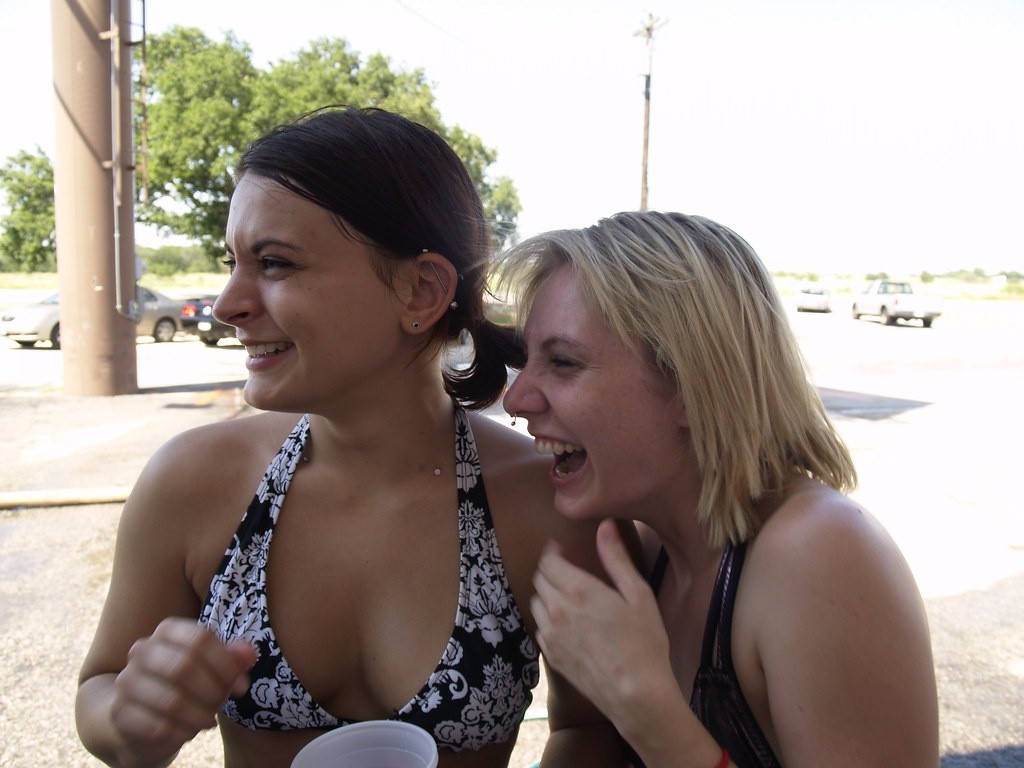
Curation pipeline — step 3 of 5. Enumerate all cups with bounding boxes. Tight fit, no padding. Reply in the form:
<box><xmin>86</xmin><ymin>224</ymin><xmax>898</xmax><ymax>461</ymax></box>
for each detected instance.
<box><xmin>290</xmin><ymin>719</ymin><xmax>439</xmax><ymax>768</ymax></box>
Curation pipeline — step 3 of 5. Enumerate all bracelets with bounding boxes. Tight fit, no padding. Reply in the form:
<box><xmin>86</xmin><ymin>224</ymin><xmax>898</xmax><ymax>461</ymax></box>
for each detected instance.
<box><xmin>714</xmin><ymin>749</ymin><xmax>729</xmax><ymax>768</ymax></box>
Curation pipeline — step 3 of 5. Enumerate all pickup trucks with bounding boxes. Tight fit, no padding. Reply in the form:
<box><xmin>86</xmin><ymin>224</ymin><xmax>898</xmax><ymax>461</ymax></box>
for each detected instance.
<box><xmin>852</xmin><ymin>279</ymin><xmax>942</xmax><ymax>328</ymax></box>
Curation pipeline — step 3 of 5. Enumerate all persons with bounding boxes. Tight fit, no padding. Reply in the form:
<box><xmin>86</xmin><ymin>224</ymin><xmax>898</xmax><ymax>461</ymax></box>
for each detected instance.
<box><xmin>75</xmin><ymin>104</ymin><xmax>645</xmax><ymax>768</ymax></box>
<box><xmin>492</xmin><ymin>212</ymin><xmax>940</xmax><ymax>768</ymax></box>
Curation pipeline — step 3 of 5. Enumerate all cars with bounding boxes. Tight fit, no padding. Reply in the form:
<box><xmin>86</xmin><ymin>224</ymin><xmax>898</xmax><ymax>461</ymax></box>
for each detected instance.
<box><xmin>797</xmin><ymin>285</ymin><xmax>829</xmax><ymax>314</ymax></box>
<box><xmin>178</xmin><ymin>295</ymin><xmax>239</xmax><ymax>346</ymax></box>
<box><xmin>0</xmin><ymin>284</ymin><xmax>186</xmax><ymax>350</ymax></box>
